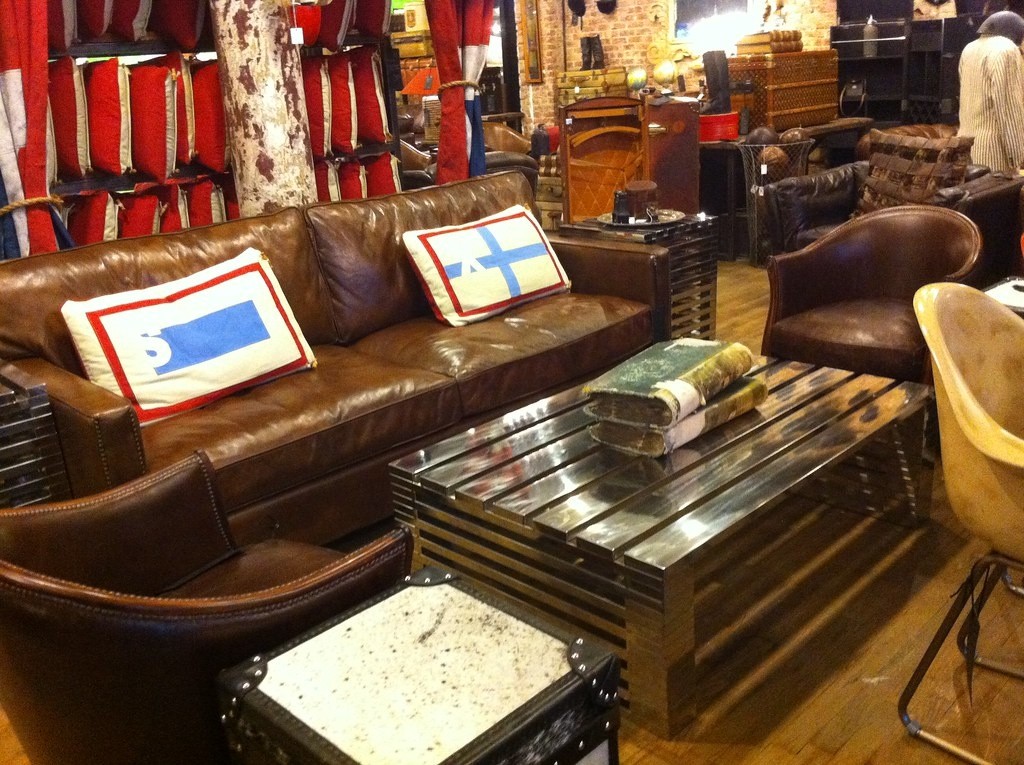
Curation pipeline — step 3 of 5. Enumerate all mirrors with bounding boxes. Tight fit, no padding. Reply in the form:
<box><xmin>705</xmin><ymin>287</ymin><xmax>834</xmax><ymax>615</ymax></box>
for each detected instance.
<box><xmin>649</xmin><ymin>0</ymin><xmax>780</xmax><ymax>70</ymax></box>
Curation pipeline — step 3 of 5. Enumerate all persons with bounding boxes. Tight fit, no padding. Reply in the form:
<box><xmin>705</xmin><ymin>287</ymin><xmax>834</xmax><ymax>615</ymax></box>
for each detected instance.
<box><xmin>957</xmin><ymin>11</ymin><xmax>1024</xmax><ymax>178</ymax></box>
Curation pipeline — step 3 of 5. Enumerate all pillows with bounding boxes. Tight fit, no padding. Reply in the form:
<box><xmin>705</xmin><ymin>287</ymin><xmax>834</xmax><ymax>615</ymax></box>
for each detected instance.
<box><xmin>847</xmin><ymin>129</ymin><xmax>975</xmax><ymax>219</ymax></box>
<box><xmin>402</xmin><ymin>203</ymin><xmax>572</xmax><ymax>327</ymax></box>
<box><xmin>1</xmin><ymin>0</ymin><xmax>401</xmax><ymax>248</ymax></box>
<box><xmin>59</xmin><ymin>247</ymin><xmax>319</xmax><ymax>428</ymax></box>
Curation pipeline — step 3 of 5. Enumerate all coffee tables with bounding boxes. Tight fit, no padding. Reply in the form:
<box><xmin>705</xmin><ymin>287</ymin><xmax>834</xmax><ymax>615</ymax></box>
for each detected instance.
<box><xmin>391</xmin><ymin>353</ymin><xmax>937</xmax><ymax>743</ymax></box>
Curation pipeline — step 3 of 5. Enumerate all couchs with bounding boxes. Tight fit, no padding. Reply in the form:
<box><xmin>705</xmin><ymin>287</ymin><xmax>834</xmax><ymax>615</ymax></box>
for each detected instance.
<box><xmin>0</xmin><ymin>172</ymin><xmax>670</xmax><ymax>545</ymax></box>
<box><xmin>763</xmin><ymin>161</ymin><xmax>1024</xmax><ymax>278</ymax></box>
<box><xmin>0</xmin><ymin>450</ymin><xmax>414</xmax><ymax>765</ymax></box>
<box><xmin>760</xmin><ymin>206</ymin><xmax>985</xmax><ymax>448</ymax></box>
<box><xmin>402</xmin><ymin>121</ymin><xmax>566</xmax><ymax>230</ymax></box>
<box><xmin>857</xmin><ymin>125</ymin><xmax>958</xmax><ymax>160</ymax></box>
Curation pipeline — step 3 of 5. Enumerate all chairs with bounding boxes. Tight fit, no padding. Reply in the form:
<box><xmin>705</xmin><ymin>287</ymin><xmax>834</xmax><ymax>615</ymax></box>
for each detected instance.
<box><xmin>897</xmin><ymin>282</ymin><xmax>1024</xmax><ymax>765</ymax></box>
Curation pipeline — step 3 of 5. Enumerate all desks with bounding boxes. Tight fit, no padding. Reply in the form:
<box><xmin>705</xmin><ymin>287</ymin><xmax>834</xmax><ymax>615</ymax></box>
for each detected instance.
<box><xmin>0</xmin><ymin>358</ymin><xmax>75</xmax><ymax>509</ymax></box>
<box><xmin>562</xmin><ymin>215</ymin><xmax>722</xmax><ymax>341</ymax></box>
<box><xmin>699</xmin><ymin>117</ymin><xmax>872</xmax><ymax>261</ymax></box>
<box><xmin>216</xmin><ymin>564</ymin><xmax>620</xmax><ymax>765</ymax></box>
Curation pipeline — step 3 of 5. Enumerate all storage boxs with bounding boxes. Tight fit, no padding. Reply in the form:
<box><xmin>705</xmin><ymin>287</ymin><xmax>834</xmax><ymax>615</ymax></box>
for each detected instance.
<box><xmin>725</xmin><ymin>50</ymin><xmax>839</xmax><ymax>132</ymax></box>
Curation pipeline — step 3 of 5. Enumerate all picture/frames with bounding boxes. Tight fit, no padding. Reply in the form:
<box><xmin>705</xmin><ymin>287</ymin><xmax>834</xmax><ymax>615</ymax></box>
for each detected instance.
<box><xmin>521</xmin><ymin>0</ymin><xmax>542</xmax><ymax>83</ymax></box>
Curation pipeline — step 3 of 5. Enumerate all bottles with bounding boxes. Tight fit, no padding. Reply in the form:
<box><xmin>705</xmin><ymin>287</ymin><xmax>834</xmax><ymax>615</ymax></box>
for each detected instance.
<box><xmin>730</xmin><ymin>81</ymin><xmax>755</xmax><ymax>135</ymax></box>
<box><xmin>863</xmin><ymin>15</ymin><xmax>878</xmax><ymax>58</ymax></box>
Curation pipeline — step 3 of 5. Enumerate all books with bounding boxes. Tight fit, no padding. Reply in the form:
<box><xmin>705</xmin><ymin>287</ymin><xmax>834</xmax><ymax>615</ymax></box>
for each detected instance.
<box><xmin>539</xmin><ymin>153</ymin><xmax>561</xmax><ymax>176</ymax></box>
<box><xmin>584</xmin><ymin>338</ymin><xmax>774</xmax><ymax>458</ymax></box>
<box><xmin>734</xmin><ymin>30</ymin><xmax>803</xmax><ymax>55</ymax></box>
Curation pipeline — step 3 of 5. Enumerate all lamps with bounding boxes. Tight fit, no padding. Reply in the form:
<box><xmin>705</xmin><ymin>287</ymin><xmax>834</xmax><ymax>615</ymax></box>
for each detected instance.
<box><xmin>399</xmin><ymin>67</ymin><xmax>443</xmax><ymax>145</ymax></box>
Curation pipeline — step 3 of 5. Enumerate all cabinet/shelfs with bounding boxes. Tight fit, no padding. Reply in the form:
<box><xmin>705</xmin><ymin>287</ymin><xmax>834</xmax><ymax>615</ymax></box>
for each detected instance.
<box><xmin>560</xmin><ymin>94</ymin><xmax>703</xmax><ymax>220</ymax></box>
<box><xmin>830</xmin><ymin>0</ymin><xmax>1024</xmax><ymax>128</ymax></box>
